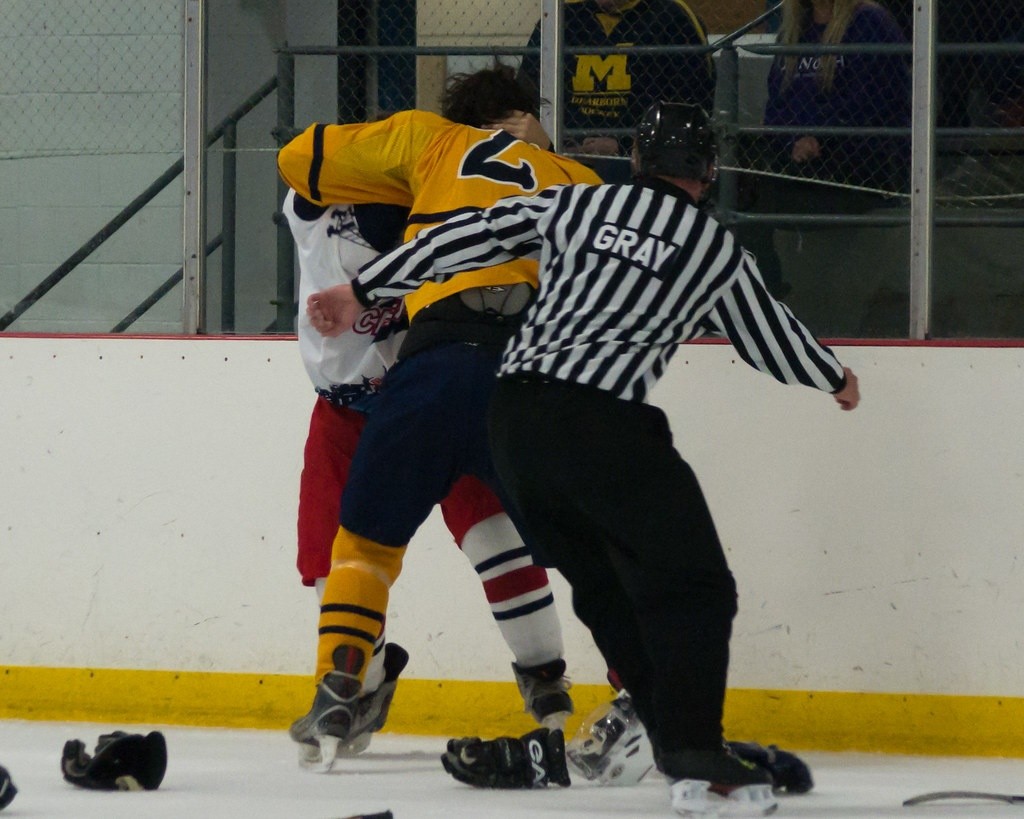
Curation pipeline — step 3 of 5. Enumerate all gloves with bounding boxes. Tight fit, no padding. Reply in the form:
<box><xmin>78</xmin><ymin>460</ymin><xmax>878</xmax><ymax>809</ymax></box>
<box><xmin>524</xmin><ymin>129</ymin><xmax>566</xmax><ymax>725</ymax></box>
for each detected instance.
<box><xmin>61</xmin><ymin>729</ymin><xmax>167</xmax><ymax>792</ymax></box>
<box><xmin>440</xmin><ymin>727</ymin><xmax>571</xmax><ymax>794</ymax></box>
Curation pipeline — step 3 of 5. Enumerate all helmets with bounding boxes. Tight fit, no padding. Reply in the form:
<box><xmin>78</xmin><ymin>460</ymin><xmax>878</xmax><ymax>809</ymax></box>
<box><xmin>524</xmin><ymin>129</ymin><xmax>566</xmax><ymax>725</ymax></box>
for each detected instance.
<box><xmin>566</xmin><ymin>692</ymin><xmax>657</xmax><ymax>787</ymax></box>
<box><xmin>631</xmin><ymin>99</ymin><xmax>718</xmax><ymax>182</ymax></box>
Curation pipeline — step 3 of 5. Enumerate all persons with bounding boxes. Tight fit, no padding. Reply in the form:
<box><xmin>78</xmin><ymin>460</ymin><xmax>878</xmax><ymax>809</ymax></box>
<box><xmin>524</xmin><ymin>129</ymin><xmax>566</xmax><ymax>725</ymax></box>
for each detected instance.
<box><xmin>277</xmin><ymin>108</ymin><xmax>577</xmax><ymax>740</ymax></box>
<box><xmin>302</xmin><ymin>101</ymin><xmax>860</xmax><ymax>793</ymax></box>
<box><xmin>277</xmin><ymin>63</ymin><xmax>602</xmax><ymax>744</ymax></box>
<box><xmin>514</xmin><ymin>1</ymin><xmax>721</xmax><ymax>185</ymax></box>
<box><xmin>756</xmin><ymin>0</ymin><xmax>912</xmax><ymax>215</ymax></box>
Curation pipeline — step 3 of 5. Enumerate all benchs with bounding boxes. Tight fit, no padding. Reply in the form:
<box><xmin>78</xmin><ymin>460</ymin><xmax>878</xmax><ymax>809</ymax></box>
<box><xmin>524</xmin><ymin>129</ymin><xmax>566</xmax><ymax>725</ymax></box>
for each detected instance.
<box><xmin>432</xmin><ymin>46</ymin><xmax>1024</xmax><ymax>258</ymax></box>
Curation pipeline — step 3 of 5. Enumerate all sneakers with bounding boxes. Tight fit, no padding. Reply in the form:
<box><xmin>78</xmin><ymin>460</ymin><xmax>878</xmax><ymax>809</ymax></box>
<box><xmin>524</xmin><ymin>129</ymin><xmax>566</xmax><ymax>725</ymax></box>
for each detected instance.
<box><xmin>288</xmin><ymin>643</ymin><xmax>363</xmax><ymax>774</ymax></box>
<box><xmin>510</xmin><ymin>662</ymin><xmax>573</xmax><ymax>733</ymax></box>
<box><xmin>649</xmin><ymin>729</ymin><xmax>778</xmax><ymax>818</ymax></box>
<box><xmin>336</xmin><ymin>643</ymin><xmax>410</xmax><ymax>759</ymax></box>
